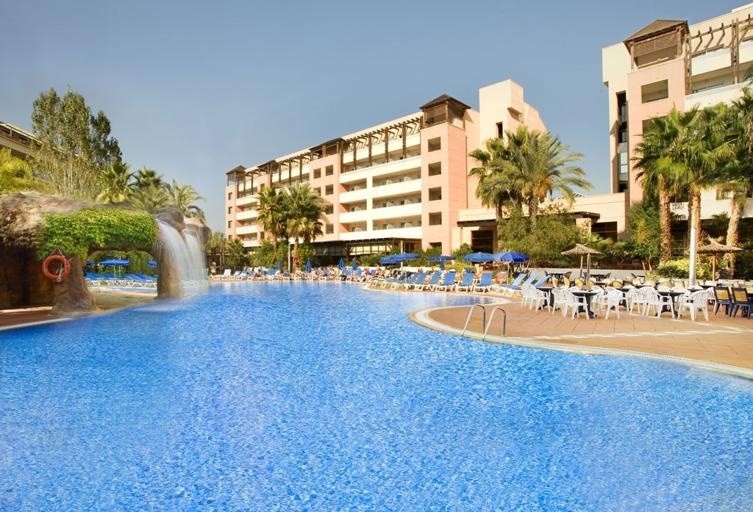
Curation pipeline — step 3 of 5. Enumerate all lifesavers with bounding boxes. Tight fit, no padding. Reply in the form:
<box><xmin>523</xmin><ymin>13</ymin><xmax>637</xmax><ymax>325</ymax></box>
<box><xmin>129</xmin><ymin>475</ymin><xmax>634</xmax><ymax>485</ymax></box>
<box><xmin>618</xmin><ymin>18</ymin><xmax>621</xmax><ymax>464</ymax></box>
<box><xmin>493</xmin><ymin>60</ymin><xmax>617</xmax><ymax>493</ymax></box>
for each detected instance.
<box><xmin>42</xmin><ymin>255</ymin><xmax>71</xmax><ymax>282</ymax></box>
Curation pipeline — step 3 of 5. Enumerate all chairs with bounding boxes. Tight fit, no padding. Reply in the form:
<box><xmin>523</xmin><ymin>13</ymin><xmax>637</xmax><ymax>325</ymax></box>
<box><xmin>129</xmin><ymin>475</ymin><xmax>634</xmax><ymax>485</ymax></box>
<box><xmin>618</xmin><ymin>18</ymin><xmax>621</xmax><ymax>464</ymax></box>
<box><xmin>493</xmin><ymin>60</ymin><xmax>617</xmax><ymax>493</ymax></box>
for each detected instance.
<box><xmin>84</xmin><ymin>272</ymin><xmax>157</xmax><ymax>289</ymax></box>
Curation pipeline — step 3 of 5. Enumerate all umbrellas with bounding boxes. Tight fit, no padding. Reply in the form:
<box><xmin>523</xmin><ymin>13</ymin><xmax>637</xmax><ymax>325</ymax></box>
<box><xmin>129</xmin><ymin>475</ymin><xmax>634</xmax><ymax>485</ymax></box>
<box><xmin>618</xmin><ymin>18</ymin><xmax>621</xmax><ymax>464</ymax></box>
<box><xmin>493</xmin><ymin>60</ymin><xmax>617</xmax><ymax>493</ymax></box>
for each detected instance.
<box><xmin>428</xmin><ymin>255</ymin><xmax>456</xmax><ymax>269</ymax></box>
<box><xmin>464</xmin><ymin>252</ymin><xmax>493</xmax><ymax>275</ymax></box>
<box><xmin>86</xmin><ymin>260</ymin><xmax>95</xmax><ymax>266</ymax></box>
<box><xmin>378</xmin><ymin>253</ymin><xmax>399</xmax><ymax>265</ymax></box>
<box><xmin>390</xmin><ymin>251</ymin><xmax>422</xmax><ymax>283</ymax></box>
<box><xmin>493</xmin><ymin>251</ymin><xmax>529</xmax><ymax>284</ymax></box>
<box><xmin>560</xmin><ymin>244</ymin><xmax>604</xmax><ymax>277</ymax></box>
<box><xmin>99</xmin><ymin>259</ymin><xmax>134</xmax><ymax>278</ymax></box>
<box><xmin>279</xmin><ymin>256</ymin><xmax>358</xmax><ymax>277</ymax></box>
<box><xmin>147</xmin><ymin>261</ymin><xmax>157</xmax><ymax>266</ymax></box>
<box><xmin>697</xmin><ymin>237</ymin><xmax>743</xmax><ymax>282</ymax></box>
